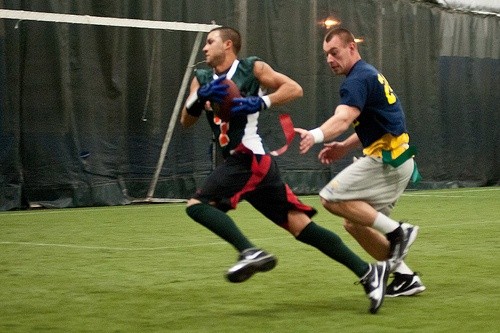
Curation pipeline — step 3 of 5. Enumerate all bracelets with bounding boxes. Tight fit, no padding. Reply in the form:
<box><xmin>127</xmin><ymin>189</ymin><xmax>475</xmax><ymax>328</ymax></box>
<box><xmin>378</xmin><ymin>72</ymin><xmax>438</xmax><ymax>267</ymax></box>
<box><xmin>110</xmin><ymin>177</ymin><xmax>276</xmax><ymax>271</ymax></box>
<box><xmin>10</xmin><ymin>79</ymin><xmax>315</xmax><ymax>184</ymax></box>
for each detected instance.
<box><xmin>310</xmin><ymin>128</ymin><xmax>324</xmax><ymax>143</ymax></box>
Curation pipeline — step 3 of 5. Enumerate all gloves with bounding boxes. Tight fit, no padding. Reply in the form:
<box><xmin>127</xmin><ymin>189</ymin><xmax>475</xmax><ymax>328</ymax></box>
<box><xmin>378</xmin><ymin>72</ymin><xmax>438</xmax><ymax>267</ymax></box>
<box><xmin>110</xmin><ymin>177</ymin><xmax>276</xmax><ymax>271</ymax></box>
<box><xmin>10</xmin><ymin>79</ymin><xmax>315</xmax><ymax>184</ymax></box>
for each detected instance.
<box><xmin>230</xmin><ymin>92</ymin><xmax>267</xmax><ymax>115</ymax></box>
<box><xmin>197</xmin><ymin>76</ymin><xmax>230</xmax><ymax>103</ymax></box>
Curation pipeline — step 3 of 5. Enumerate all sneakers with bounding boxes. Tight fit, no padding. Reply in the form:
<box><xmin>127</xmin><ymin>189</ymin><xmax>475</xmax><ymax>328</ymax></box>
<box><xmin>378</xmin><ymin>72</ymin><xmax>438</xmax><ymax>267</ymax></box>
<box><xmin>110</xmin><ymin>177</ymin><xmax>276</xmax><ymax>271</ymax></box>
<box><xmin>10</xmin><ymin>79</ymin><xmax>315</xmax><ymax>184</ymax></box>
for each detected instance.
<box><xmin>354</xmin><ymin>261</ymin><xmax>390</xmax><ymax>313</ymax></box>
<box><xmin>385</xmin><ymin>271</ymin><xmax>425</xmax><ymax>298</ymax></box>
<box><xmin>227</xmin><ymin>248</ymin><xmax>277</xmax><ymax>283</ymax></box>
<box><xmin>384</xmin><ymin>222</ymin><xmax>419</xmax><ymax>272</ymax></box>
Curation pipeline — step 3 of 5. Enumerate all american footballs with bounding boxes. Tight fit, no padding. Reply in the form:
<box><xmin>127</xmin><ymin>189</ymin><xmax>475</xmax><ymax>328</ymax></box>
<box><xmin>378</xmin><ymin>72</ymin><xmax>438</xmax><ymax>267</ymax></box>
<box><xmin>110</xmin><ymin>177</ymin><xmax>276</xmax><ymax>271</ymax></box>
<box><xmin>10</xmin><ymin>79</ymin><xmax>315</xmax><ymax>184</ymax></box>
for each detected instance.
<box><xmin>211</xmin><ymin>79</ymin><xmax>242</xmax><ymax>122</ymax></box>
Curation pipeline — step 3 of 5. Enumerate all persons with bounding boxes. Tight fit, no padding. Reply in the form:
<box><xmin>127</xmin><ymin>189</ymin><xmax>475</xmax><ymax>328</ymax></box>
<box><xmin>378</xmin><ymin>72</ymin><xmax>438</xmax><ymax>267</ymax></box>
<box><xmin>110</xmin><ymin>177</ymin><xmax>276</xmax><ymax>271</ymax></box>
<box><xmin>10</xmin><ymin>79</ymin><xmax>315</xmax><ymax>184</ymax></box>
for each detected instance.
<box><xmin>180</xmin><ymin>27</ymin><xmax>390</xmax><ymax>313</ymax></box>
<box><xmin>293</xmin><ymin>28</ymin><xmax>425</xmax><ymax>298</ymax></box>
